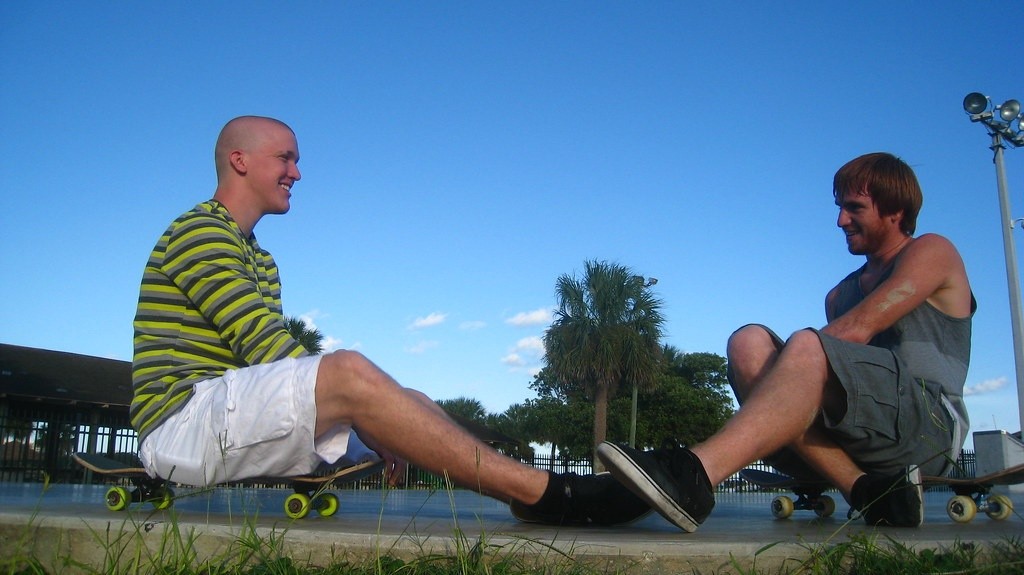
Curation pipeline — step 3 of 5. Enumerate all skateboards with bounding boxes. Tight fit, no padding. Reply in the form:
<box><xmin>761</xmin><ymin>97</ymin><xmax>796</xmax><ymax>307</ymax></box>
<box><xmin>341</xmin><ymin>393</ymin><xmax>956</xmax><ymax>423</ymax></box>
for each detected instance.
<box><xmin>738</xmin><ymin>463</ymin><xmax>1024</xmax><ymax>523</ymax></box>
<box><xmin>67</xmin><ymin>447</ymin><xmax>392</xmax><ymax>519</ymax></box>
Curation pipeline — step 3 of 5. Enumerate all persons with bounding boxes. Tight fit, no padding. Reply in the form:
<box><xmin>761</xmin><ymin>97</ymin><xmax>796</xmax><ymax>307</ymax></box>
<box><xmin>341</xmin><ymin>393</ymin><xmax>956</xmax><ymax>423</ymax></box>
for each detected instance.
<box><xmin>596</xmin><ymin>153</ymin><xmax>978</xmax><ymax>533</ymax></box>
<box><xmin>129</xmin><ymin>116</ymin><xmax>655</xmax><ymax>528</ymax></box>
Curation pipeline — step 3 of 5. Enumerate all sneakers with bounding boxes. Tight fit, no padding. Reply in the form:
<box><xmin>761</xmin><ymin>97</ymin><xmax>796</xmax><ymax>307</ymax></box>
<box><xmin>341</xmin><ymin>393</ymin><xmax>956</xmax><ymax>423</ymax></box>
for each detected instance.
<box><xmin>596</xmin><ymin>441</ymin><xmax>715</xmax><ymax>533</ymax></box>
<box><xmin>509</xmin><ymin>469</ymin><xmax>655</xmax><ymax>526</ymax></box>
<box><xmin>847</xmin><ymin>464</ymin><xmax>923</xmax><ymax>527</ymax></box>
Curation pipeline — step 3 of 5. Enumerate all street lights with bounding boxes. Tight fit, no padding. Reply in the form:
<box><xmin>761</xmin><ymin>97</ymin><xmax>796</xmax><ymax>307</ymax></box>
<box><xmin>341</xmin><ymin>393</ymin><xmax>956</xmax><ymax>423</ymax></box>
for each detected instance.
<box><xmin>962</xmin><ymin>92</ymin><xmax>1024</xmax><ymax>442</ymax></box>
